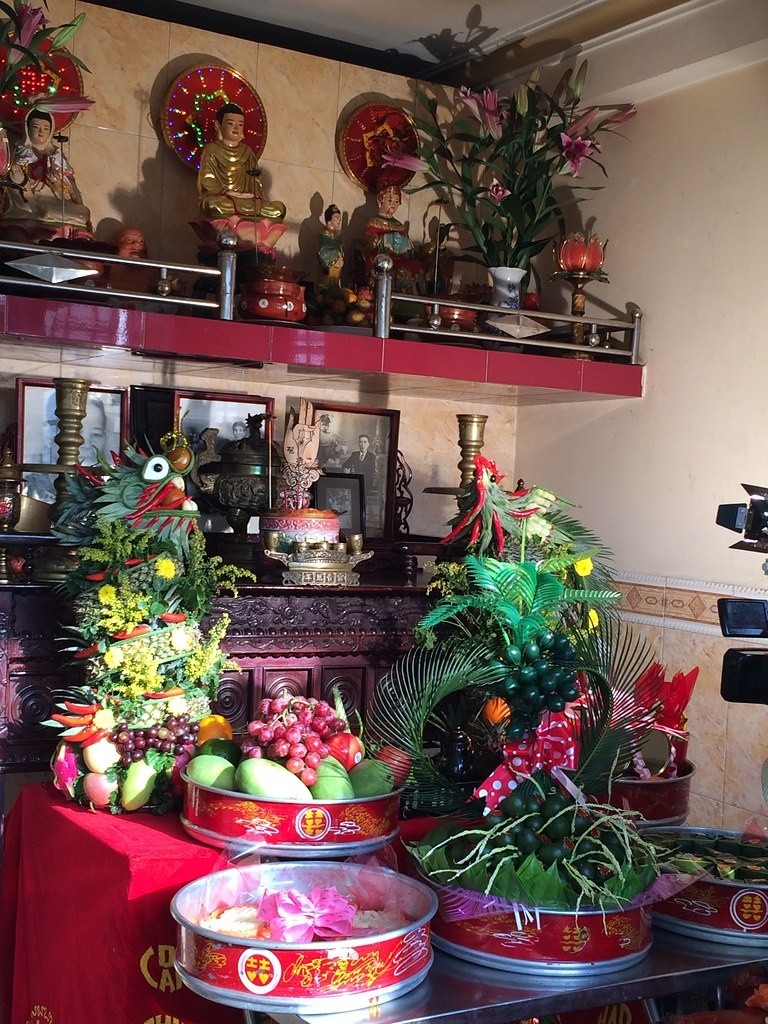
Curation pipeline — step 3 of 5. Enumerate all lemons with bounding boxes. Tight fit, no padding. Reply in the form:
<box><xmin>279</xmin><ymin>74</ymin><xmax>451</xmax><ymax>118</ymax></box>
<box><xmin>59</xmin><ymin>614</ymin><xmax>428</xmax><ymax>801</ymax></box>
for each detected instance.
<box><xmin>194</xmin><ymin>714</ymin><xmax>241</xmax><ymax>767</ymax></box>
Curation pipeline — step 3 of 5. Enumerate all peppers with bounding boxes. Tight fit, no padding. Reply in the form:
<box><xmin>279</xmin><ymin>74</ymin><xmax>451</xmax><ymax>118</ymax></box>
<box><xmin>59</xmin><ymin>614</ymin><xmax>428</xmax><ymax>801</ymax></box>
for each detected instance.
<box><xmin>50</xmin><ymin>448</ymin><xmax>194</xmax><ymax>748</ymax></box>
<box><xmin>439</xmin><ymin>455</ymin><xmax>540</xmax><ymax>558</ymax></box>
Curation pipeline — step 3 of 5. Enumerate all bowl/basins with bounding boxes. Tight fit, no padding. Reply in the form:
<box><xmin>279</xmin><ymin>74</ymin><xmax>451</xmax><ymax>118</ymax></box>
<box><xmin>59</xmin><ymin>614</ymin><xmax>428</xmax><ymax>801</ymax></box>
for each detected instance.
<box><xmin>313</xmin><ymin>326</ymin><xmax>373</xmax><ymax>336</ymax></box>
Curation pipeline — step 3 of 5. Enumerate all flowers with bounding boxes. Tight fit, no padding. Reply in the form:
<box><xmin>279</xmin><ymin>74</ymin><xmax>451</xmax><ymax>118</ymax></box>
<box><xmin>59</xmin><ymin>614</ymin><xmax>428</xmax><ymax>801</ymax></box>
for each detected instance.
<box><xmin>0</xmin><ymin>0</ymin><xmax>96</xmax><ymax>192</ymax></box>
<box><xmin>380</xmin><ymin>57</ymin><xmax>638</xmax><ymax>306</ymax></box>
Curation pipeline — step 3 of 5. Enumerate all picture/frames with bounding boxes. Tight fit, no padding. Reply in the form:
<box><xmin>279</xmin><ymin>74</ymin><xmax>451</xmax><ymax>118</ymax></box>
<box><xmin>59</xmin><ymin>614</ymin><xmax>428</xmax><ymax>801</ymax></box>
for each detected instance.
<box><xmin>310</xmin><ymin>403</ymin><xmax>400</xmax><ymax>543</ymax></box>
<box><xmin>173</xmin><ymin>389</ymin><xmax>276</xmax><ymax>533</ymax></box>
<box><xmin>129</xmin><ymin>385</ymin><xmax>174</xmax><ymax>457</ymax></box>
<box><xmin>312</xmin><ymin>472</ymin><xmax>366</xmax><ymax>544</ymax></box>
<box><xmin>16</xmin><ymin>378</ymin><xmax>130</xmax><ymax>536</ymax></box>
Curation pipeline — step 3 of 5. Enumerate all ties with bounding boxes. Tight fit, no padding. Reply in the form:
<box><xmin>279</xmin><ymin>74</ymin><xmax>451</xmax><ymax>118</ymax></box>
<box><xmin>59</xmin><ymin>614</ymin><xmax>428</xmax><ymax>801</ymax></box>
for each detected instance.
<box><xmin>360</xmin><ymin>455</ymin><xmax>364</xmax><ymax>462</ymax></box>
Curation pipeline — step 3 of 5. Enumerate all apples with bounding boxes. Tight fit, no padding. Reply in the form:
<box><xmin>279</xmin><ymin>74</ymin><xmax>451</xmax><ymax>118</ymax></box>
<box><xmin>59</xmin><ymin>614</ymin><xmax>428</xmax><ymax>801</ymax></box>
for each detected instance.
<box><xmin>375</xmin><ymin>744</ymin><xmax>412</xmax><ymax>789</ymax></box>
<box><xmin>82</xmin><ymin>736</ymin><xmax>122</xmax><ymax>806</ymax></box>
<box><xmin>325</xmin><ymin>732</ymin><xmax>366</xmax><ymax>769</ymax></box>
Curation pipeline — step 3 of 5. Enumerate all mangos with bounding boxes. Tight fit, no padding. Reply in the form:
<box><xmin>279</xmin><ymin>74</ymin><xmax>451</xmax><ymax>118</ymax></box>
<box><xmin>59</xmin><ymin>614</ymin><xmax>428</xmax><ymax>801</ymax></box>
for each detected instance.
<box><xmin>119</xmin><ymin>758</ymin><xmax>158</xmax><ymax>811</ymax></box>
<box><xmin>185</xmin><ymin>751</ymin><xmax>394</xmax><ymax>801</ymax></box>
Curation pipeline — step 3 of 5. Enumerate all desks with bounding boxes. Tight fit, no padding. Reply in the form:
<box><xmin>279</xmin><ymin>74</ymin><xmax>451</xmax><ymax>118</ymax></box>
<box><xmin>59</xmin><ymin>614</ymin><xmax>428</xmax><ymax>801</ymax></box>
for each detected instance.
<box><xmin>0</xmin><ymin>779</ymin><xmax>478</xmax><ymax>1024</ymax></box>
<box><xmin>241</xmin><ymin>926</ymin><xmax>768</xmax><ymax>1023</ymax></box>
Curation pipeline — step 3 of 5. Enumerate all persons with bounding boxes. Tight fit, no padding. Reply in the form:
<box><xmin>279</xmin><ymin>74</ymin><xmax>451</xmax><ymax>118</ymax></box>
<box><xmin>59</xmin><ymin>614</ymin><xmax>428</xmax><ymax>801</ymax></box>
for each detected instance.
<box><xmin>231</xmin><ymin>420</ymin><xmax>247</xmax><ymax>440</ymax></box>
<box><xmin>0</xmin><ymin>107</ymin><xmax>92</xmax><ymax>228</ymax></box>
<box><xmin>360</xmin><ymin>178</ymin><xmax>428</xmax><ymax>295</ymax></box>
<box><xmin>197</xmin><ymin>103</ymin><xmax>287</xmax><ymax>219</ymax></box>
<box><xmin>317</xmin><ymin>414</ymin><xmax>377</xmax><ymax>528</ymax></box>
<box><xmin>316</xmin><ymin>204</ymin><xmax>346</xmax><ymax>287</ymax></box>
<box><xmin>24</xmin><ymin>392</ymin><xmax>107</xmax><ymax>502</ymax></box>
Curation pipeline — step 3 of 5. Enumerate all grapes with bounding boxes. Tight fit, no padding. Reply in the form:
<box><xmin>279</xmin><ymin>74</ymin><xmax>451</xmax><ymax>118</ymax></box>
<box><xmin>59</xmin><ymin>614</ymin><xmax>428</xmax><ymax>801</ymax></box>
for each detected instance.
<box><xmin>239</xmin><ymin>688</ymin><xmax>347</xmax><ymax>786</ymax></box>
<box><xmin>109</xmin><ymin>713</ymin><xmax>200</xmax><ymax>764</ymax></box>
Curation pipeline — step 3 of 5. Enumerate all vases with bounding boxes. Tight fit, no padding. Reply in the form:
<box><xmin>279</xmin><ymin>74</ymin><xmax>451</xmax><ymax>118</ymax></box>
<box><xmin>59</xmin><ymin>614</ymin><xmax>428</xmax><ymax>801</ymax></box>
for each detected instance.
<box><xmin>485</xmin><ymin>267</ymin><xmax>529</xmax><ymax>351</ymax></box>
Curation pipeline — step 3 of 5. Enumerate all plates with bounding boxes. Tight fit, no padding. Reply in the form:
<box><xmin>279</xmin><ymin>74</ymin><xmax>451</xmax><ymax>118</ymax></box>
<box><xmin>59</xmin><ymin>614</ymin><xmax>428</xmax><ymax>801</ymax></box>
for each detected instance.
<box><xmin>436</xmin><ymin>343</ymin><xmax>484</xmax><ymax>348</ymax></box>
<box><xmin>236</xmin><ymin>318</ymin><xmax>309</xmax><ymax>327</ymax></box>
